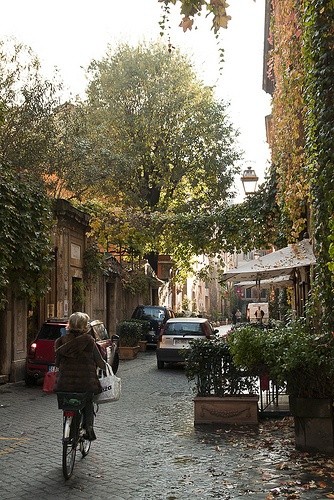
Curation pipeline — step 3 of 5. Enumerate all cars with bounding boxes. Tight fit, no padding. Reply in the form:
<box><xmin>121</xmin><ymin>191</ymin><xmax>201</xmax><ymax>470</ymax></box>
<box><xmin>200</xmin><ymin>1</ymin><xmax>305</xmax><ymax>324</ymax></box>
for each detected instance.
<box><xmin>156</xmin><ymin>317</ymin><xmax>219</xmax><ymax>369</ymax></box>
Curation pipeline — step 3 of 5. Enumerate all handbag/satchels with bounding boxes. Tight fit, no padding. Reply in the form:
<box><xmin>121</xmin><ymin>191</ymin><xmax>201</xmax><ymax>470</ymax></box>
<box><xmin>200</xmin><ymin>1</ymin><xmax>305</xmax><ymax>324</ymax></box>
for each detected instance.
<box><xmin>43</xmin><ymin>372</ymin><xmax>58</xmax><ymax>392</ymax></box>
<box><xmin>93</xmin><ymin>363</ymin><xmax>122</xmax><ymax>402</ymax></box>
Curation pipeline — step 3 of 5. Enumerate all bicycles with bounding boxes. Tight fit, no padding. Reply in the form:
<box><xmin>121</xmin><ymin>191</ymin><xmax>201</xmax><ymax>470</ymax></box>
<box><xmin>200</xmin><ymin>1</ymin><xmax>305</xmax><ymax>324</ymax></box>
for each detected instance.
<box><xmin>61</xmin><ymin>396</ymin><xmax>94</xmax><ymax>479</ymax></box>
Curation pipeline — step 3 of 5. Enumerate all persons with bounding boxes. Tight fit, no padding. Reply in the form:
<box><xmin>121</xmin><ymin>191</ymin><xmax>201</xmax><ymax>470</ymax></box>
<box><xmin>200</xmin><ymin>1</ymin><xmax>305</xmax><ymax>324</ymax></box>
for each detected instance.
<box><xmin>54</xmin><ymin>312</ymin><xmax>107</xmax><ymax>441</ymax></box>
<box><xmin>235</xmin><ymin>309</ymin><xmax>241</xmax><ymax>322</ymax></box>
<box><xmin>255</xmin><ymin>306</ymin><xmax>264</xmax><ymax>322</ymax></box>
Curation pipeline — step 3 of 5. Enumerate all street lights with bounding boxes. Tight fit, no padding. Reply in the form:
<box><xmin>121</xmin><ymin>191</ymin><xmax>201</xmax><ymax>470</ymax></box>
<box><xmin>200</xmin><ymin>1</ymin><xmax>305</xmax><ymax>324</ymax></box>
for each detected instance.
<box><xmin>241</xmin><ymin>166</ymin><xmax>258</xmax><ymax>211</ymax></box>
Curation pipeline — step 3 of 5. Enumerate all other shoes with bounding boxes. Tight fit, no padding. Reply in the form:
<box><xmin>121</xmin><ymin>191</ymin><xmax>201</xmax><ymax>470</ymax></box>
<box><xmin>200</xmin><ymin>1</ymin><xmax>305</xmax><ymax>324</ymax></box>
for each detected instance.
<box><xmin>86</xmin><ymin>427</ymin><xmax>97</xmax><ymax>441</ymax></box>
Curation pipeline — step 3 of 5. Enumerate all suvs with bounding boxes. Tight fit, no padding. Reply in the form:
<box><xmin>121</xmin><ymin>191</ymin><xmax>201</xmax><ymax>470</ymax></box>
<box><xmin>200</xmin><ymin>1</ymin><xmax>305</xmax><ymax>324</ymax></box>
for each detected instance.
<box><xmin>23</xmin><ymin>318</ymin><xmax>120</xmax><ymax>390</ymax></box>
<box><xmin>131</xmin><ymin>305</ymin><xmax>176</xmax><ymax>346</ymax></box>
